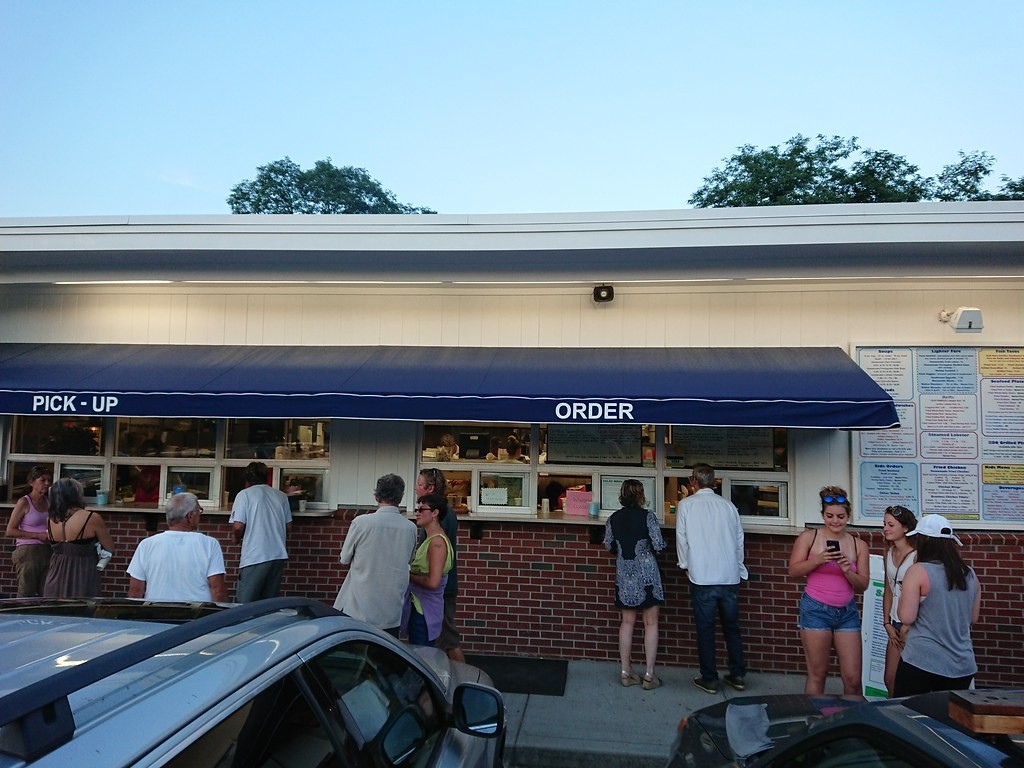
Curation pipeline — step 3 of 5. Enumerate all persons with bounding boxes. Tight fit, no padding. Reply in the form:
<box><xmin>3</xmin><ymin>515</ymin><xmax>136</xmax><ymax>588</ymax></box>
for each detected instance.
<box><xmin>434</xmin><ymin>437</ymin><xmax>468</xmax><ymax>504</ymax></box>
<box><xmin>893</xmin><ymin>515</ymin><xmax>981</xmax><ymax>698</ymax></box>
<box><xmin>788</xmin><ymin>488</ymin><xmax>869</xmax><ymax>696</ymax></box>
<box><xmin>5</xmin><ymin>466</ymin><xmax>51</xmax><ymax>598</ymax></box>
<box><xmin>334</xmin><ymin>474</ymin><xmax>418</xmax><ymax>639</ymax></box>
<box><xmin>135</xmin><ymin>465</ymin><xmax>183</xmax><ymax>507</ymax></box>
<box><xmin>401</xmin><ymin>496</ymin><xmax>455</xmax><ymax>647</ymax></box>
<box><xmin>126</xmin><ymin>493</ymin><xmax>228</xmax><ymax>603</ymax></box>
<box><xmin>676</xmin><ymin>463</ymin><xmax>748</xmax><ymax>694</ymax></box>
<box><xmin>604</xmin><ymin>479</ymin><xmax>668</xmax><ymax>689</ymax></box>
<box><xmin>882</xmin><ymin>505</ymin><xmax>917</xmax><ymax>698</ymax></box>
<box><xmin>229</xmin><ymin>463</ymin><xmax>294</xmax><ymax>604</ymax></box>
<box><xmin>487</xmin><ymin>437</ymin><xmax>523</xmax><ymax>498</ymax></box>
<box><xmin>230</xmin><ymin>437</ymin><xmax>262</xmax><ymax>501</ymax></box>
<box><xmin>43</xmin><ymin>478</ymin><xmax>114</xmax><ymax>596</ymax></box>
<box><xmin>415</xmin><ymin>470</ymin><xmax>465</xmax><ymax>664</ymax></box>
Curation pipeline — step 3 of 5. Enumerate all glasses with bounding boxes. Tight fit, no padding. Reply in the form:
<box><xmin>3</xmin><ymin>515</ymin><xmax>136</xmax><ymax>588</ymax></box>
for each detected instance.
<box><xmin>885</xmin><ymin>506</ymin><xmax>903</xmax><ymax>516</ymax></box>
<box><xmin>822</xmin><ymin>495</ymin><xmax>850</xmax><ymax>505</ymax></box>
<box><xmin>689</xmin><ymin>476</ymin><xmax>698</xmax><ymax>481</ymax></box>
<box><xmin>186</xmin><ymin>507</ymin><xmax>204</xmax><ymax>517</ymax></box>
<box><xmin>432</xmin><ymin>468</ymin><xmax>436</xmax><ymax>479</ymax></box>
<box><xmin>415</xmin><ymin>507</ymin><xmax>432</xmax><ymax>513</ymax></box>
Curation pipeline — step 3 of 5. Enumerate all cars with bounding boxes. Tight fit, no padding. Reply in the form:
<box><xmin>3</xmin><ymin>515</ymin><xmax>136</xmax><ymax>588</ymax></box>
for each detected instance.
<box><xmin>663</xmin><ymin>685</ymin><xmax>1024</xmax><ymax>768</ymax></box>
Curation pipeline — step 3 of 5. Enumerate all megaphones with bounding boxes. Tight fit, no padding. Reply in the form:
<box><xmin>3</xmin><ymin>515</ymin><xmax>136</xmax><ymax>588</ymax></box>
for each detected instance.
<box><xmin>594</xmin><ymin>286</ymin><xmax>614</xmax><ymax>302</ymax></box>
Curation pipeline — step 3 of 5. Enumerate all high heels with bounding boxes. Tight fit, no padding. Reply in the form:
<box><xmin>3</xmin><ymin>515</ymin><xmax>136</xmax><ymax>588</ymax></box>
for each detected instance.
<box><xmin>622</xmin><ymin>669</ymin><xmax>642</xmax><ymax>686</ymax></box>
<box><xmin>641</xmin><ymin>672</ymin><xmax>662</xmax><ymax>690</ymax></box>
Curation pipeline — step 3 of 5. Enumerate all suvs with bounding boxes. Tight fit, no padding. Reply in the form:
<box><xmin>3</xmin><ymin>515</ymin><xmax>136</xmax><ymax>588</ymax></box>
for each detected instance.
<box><xmin>0</xmin><ymin>595</ymin><xmax>510</xmax><ymax>768</ymax></box>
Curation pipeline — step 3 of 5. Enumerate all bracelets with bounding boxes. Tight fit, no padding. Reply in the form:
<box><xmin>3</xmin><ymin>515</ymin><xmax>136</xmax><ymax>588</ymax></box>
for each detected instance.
<box><xmin>883</xmin><ymin>623</ymin><xmax>891</xmax><ymax>626</ymax></box>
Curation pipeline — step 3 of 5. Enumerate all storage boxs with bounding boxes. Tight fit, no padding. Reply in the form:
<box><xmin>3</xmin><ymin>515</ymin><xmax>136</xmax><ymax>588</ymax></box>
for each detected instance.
<box><xmin>423</xmin><ymin>450</ymin><xmax>437</xmax><ymax>458</ymax></box>
<box><xmin>426</xmin><ymin>448</ymin><xmax>437</xmax><ymax>451</ymax></box>
<box><xmin>665</xmin><ymin>502</ymin><xmax>671</xmax><ymax>517</ymax></box>
<box><xmin>947</xmin><ymin>689</ymin><xmax>1024</xmax><ymax>735</ymax></box>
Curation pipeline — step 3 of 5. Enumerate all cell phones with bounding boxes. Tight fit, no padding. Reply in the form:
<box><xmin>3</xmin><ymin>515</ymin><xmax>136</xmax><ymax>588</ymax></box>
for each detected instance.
<box><xmin>827</xmin><ymin>539</ymin><xmax>841</xmax><ymax>559</ymax></box>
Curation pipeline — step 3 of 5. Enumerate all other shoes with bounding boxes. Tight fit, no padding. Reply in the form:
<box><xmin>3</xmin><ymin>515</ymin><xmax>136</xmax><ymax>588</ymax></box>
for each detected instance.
<box><xmin>693</xmin><ymin>676</ymin><xmax>716</xmax><ymax>695</ymax></box>
<box><xmin>723</xmin><ymin>674</ymin><xmax>746</xmax><ymax>690</ymax></box>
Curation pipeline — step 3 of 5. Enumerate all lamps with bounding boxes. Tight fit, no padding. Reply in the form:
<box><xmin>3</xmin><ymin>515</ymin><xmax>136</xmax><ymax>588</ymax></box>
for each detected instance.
<box><xmin>938</xmin><ymin>307</ymin><xmax>983</xmax><ymax>334</ymax></box>
<box><xmin>591</xmin><ymin>282</ymin><xmax>615</xmax><ymax>305</ymax></box>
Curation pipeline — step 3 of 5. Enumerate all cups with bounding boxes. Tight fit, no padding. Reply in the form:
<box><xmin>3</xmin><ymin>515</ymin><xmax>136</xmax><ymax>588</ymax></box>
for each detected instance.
<box><xmin>665</xmin><ymin>501</ymin><xmax>679</xmax><ymax>516</ymax></box>
<box><xmin>95</xmin><ymin>490</ymin><xmax>109</xmax><ymax>505</ymax></box>
<box><xmin>514</xmin><ymin>498</ymin><xmax>522</xmax><ymax>506</ymax></box>
<box><xmin>96</xmin><ymin>555</ymin><xmax>112</xmax><ymax>571</ymax></box>
<box><xmin>586</xmin><ymin>502</ymin><xmax>599</xmax><ymax>516</ymax></box>
<box><xmin>560</xmin><ymin>498</ymin><xmax>566</xmax><ymax>512</ymax></box>
<box><xmin>298</xmin><ymin>500</ymin><xmax>307</xmax><ymax>512</ymax></box>
<box><xmin>275</xmin><ymin>446</ymin><xmax>289</xmax><ymax>459</ymax></box>
<box><xmin>446</xmin><ymin>495</ymin><xmax>471</xmax><ymax>513</ymax></box>
<box><xmin>542</xmin><ymin>499</ymin><xmax>549</xmax><ymax>512</ymax></box>
<box><xmin>299</xmin><ymin>426</ymin><xmax>313</xmax><ymax>443</ymax></box>
<box><xmin>224</xmin><ymin>491</ymin><xmax>229</xmax><ymax>509</ymax></box>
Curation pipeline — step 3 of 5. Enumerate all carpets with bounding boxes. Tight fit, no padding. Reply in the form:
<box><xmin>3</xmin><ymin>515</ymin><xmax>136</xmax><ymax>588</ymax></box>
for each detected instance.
<box><xmin>462</xmin><ymin>654</ymin><xmax>569</xmax><ymax>697</ymax></box>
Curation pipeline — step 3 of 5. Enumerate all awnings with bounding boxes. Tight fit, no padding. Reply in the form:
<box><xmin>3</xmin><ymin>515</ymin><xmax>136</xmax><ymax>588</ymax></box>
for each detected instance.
<box><xmin>1</xmin><ymin>342</ymin><xmax>901</xmax><ymax>431</ymax></box>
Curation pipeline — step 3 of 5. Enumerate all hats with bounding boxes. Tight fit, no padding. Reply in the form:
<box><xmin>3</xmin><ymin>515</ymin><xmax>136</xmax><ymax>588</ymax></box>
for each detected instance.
<box><xmin>906</xmin><ymin>514</ymin><xmax>963</xmax><ymax>546</ymax></box>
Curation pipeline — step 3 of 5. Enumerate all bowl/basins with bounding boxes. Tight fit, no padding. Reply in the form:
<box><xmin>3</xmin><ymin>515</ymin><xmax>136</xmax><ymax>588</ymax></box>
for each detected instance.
<box><xmin>161</xmin><ymin>445</ymin><xmax>215</xmax><ymax>457</ymax></box>
